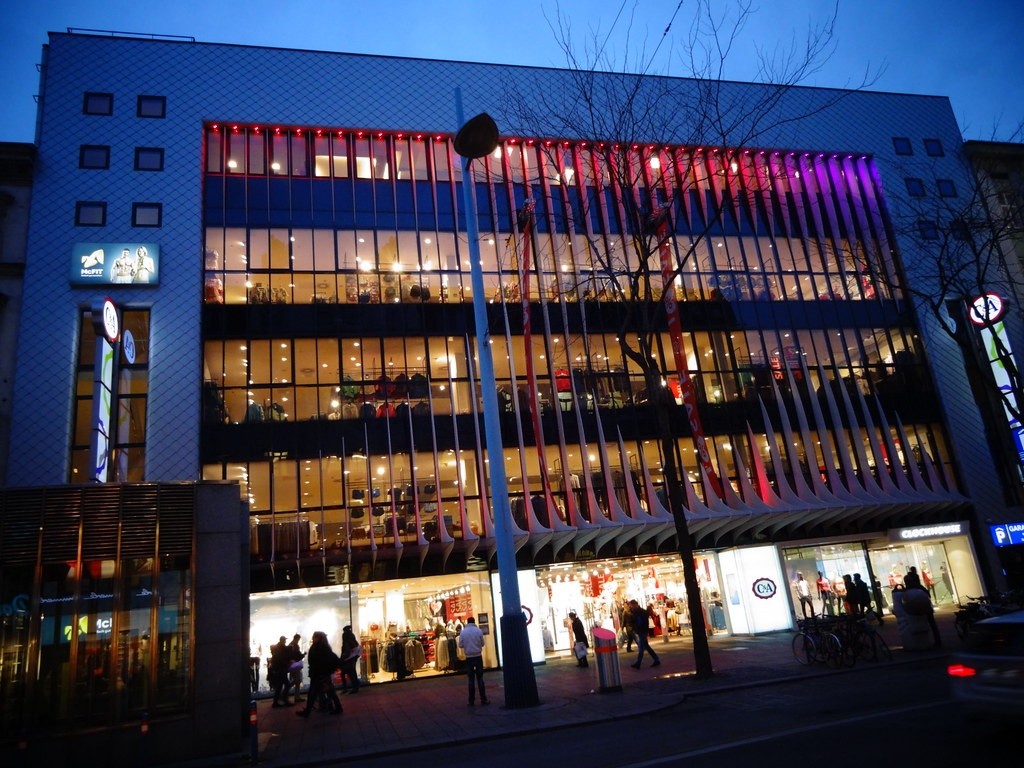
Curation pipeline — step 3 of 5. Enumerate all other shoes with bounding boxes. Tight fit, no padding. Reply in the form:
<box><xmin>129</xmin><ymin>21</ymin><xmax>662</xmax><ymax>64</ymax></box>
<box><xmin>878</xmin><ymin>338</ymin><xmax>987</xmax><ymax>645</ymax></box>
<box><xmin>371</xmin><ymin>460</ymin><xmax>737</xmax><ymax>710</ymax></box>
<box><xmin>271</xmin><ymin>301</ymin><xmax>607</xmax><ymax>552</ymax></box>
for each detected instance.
<box><xmin>316</xmin><ymin>706</ymin><xmax>329</xmax><ymax>714</ymax></box>
<box><xmin>340</xmin><ymin>688</ymin><xmax>348</xmax><ymax>694</ymax></box>
<box><xmin>630</xmin><ymin>664</ymin><xmax>640</xmax><ymax>669</ymax></box>
<box><xmin>349</xmin><ymin>689</ymin><xmax>358</xmax><ymax>693</ymax></box>
<box><xmin>878</xmin><ymin>620</ymin><xmax>883</xmax><ymax>626</ymax></box>
<box><xmin>481</xmin><ymin>699</ymin><xmax>490</xmax><ymax>705</ymax></box>
<box><xmin>283</xmin><ymin>700</ymin><xmax>294</xmax><ymax>707</ymax></box>
<box><xmin>294</xmin><ymin>698</ymin><xmax>306</xmax><ymax>703</ymax></box>
<box><xmin>271</xmin><ymin>701</ymin><xmax>281</xmax><ymax>707</ymax></box>
<box><xmin>329</xmin><ymin>710</ymin><xmax>343</xmax><ymax>715</ymax></box>
<box><xmin>576</xmin><ymin>661</ymin><xmax>588</xmax><ymax>667</ymax></box>
<box><xmin>466</xmin><ymin>703</ymin><xmax>475</xmax><ymax>708</ymax></box>
<box><xmin>627</xmin><ymin>648</ymin><xmax>634</xmax><ymax>652</ymax></box>
<box><xmin>296</xmin><ymin>707</ymin><xmax>309</xmax><ymax>719</ymax></box>
<box><xmin>651</xmin><ymin>660</ymin><xmax>660</xmax><ymax>666</ymax></box>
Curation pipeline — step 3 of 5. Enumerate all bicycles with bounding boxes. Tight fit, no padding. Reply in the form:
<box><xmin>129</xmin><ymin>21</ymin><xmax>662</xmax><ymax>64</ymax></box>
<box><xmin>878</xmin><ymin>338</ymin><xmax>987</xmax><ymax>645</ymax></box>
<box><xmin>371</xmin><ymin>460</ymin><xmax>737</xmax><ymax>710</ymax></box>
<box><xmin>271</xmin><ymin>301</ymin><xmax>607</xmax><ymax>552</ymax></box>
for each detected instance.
<box><xmin>792</xmin><ymin>604</ymin><xmax>894</xmax><ymax>670</ymax></box>
<box><xmin>954</xmin><ymin>588</ymin><xmax>1024</xmax><ymax>641</ymax></box>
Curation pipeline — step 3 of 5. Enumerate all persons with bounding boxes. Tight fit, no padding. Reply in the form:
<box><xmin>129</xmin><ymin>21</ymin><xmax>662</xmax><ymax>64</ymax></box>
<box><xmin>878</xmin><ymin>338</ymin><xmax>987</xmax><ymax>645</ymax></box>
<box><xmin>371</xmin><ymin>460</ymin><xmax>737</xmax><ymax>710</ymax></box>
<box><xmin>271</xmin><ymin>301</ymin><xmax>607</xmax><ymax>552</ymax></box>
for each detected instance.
<box><xmin>816</xmin><ymin>570</ymin><xmax>834</xmax><ymax>618</ymax></box>
<box><xmin>792</xmin><ymin>572</ymin><xmax>814</xmax><ymax>619</ymax></box>
<box><xmin>842</xmin><ymin>561</ymin><xmax>954</xmax><ymax>648</ymax></box>
<box><xmin>266</xmin><ymin>625</ymin><xmax>362</xmax><ymax>719</ymax></box>
<box><xmin>888</xmin><ymin>564</ymin><xmax>905</xmax><ymax>589</ymax></box>
<box><xmin>459</xmin><ymin>617</ymin><xmax>491</xmax><ymax>706</ymax></box>
<box><xmin>832</xmin><ymin>569</ymin><xmax>847</xmax><ymax>614</ymax></box>
<box><xmin>569</xmin><ymin>592</ymin><xmax>722</xmax><ymax>669</ymax></box>
<box><xmin>558</xmin><ymin>471</ymin><xmax>582</xmax><ymax>500</ymax></box>
<box><xmin>922</xmin><ymin>561</ymin><xmax>937</xmax><ymax>604</ymax></box>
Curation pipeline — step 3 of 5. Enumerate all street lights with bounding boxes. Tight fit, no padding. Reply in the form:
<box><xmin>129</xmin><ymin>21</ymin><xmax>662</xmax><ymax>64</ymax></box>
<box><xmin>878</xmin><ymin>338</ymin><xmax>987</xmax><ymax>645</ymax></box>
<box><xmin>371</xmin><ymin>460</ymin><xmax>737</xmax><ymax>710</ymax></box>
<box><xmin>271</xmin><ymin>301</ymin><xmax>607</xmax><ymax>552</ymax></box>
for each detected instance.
<box><xmin>452</xmin><ymin>83</ymin><xmax>543</xmax><ymax>712</ymax></box>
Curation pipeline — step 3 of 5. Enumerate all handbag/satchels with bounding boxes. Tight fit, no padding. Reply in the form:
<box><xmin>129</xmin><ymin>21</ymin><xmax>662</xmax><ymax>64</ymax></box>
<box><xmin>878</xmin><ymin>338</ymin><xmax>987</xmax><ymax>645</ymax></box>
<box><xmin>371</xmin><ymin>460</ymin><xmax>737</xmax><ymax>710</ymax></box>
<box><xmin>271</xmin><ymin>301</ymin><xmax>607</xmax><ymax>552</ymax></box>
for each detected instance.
<box><xmin>574</xmin><ymin>641</ymin><xmax>588</xmax><ymax>659</ymax></box>
<box><xmin>288</xmin><ymin>660</ymin><xmax>304</xmax><ymax>671</ymax></box>
<box><xmin>343</xmin><ymin>644</ymin><xmax>361</xmax><ymax>662</ymax></box>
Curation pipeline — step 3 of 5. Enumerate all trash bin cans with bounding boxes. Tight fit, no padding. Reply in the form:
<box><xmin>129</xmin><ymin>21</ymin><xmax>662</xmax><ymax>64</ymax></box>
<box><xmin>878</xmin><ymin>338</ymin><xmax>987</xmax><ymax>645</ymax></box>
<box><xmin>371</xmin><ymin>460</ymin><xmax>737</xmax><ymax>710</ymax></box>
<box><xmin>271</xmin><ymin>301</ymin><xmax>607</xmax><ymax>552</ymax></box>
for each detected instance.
<box><xmin>892</xmin><ymin>584</ymin><xmax>931</xmax><ymax>650</ymax></box>
<box><xmin>593</xmin><ymin>627</ymin><xmax>623</xmax><ymax>692</ymax></box>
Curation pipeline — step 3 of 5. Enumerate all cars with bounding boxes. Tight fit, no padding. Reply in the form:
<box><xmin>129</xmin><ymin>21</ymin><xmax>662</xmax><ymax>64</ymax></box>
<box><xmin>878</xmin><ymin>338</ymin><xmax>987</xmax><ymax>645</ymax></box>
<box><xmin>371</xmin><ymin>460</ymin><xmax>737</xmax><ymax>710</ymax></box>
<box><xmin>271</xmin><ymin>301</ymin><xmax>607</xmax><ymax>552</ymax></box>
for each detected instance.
<box><xmin>947</xmin><ymin>610</ymin><xmax>1024</xmax><ymax>720</ymax></box>
